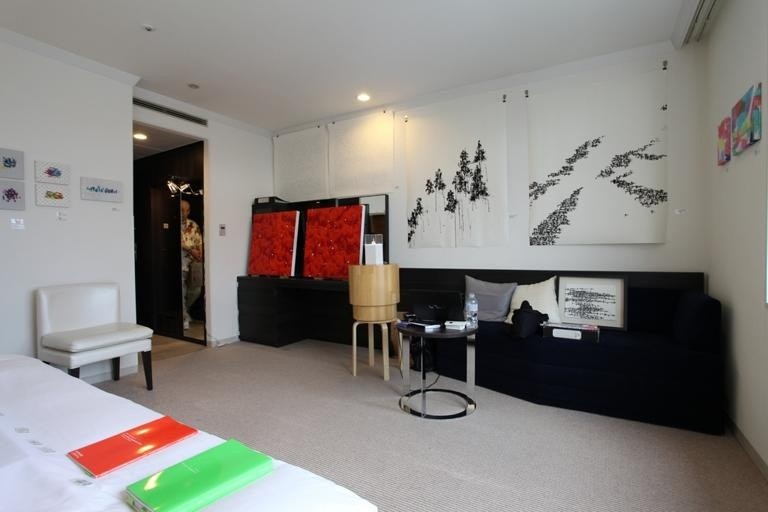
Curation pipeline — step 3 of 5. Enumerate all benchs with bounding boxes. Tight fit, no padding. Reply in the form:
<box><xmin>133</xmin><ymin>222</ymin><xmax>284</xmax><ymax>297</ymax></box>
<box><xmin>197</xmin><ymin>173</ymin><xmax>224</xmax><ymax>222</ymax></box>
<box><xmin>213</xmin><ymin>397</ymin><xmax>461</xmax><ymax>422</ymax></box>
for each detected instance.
<box><xmin>36</xmin><ymin>281</ymin><xmax>154</xmax><ymax>390</ymax></box>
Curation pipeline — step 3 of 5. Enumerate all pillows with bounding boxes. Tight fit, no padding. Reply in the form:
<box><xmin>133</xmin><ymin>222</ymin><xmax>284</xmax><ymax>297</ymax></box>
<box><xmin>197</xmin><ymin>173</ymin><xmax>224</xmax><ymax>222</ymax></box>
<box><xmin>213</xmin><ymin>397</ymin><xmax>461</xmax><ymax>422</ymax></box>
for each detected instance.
<box><xmin>463</xmin><ymin>275</ymin><xmax>517</xmax><ymax>323</ymax></box>
<box><xmin>505</xmin><ymin>275</ymin><xmax>561</xmax><ymax>324</ymax></box>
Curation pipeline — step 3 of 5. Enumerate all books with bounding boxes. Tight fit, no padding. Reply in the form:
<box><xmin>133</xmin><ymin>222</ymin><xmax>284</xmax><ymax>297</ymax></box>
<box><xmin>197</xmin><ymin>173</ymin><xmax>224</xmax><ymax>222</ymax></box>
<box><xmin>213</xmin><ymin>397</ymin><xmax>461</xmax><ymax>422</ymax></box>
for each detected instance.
<box><xmin>410</xmin><ymin>321</ymin><xmax>440</xmax><ymax>329</ymax></box>
<box><xmin>127</xmin><ymin>436</ymin><xmax>274</xmax><ymax>512</ymax></box>
<box><xmin>67</xmin><ymin>416</ymin><xmax>199</xmax><ymax>480</ymax></box>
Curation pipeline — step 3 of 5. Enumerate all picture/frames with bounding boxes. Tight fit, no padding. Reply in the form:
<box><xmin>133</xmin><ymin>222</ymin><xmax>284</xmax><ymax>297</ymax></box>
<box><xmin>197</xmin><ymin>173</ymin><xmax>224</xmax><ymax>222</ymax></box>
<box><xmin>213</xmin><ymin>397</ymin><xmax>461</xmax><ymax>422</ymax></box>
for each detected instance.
<box><xmin>555</xmin><ymin>274</ymin><xmax>627</xmax><ymax>331</ymax></box>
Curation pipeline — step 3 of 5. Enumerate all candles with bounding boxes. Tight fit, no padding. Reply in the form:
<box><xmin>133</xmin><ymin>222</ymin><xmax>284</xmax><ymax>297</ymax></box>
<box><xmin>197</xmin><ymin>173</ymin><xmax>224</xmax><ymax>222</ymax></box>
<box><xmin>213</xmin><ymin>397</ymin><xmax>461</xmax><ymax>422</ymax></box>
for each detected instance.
<box><xmin>365</xmin><ymin>241</ymin><xmax>382</xmax><ymax>265</ymax></box>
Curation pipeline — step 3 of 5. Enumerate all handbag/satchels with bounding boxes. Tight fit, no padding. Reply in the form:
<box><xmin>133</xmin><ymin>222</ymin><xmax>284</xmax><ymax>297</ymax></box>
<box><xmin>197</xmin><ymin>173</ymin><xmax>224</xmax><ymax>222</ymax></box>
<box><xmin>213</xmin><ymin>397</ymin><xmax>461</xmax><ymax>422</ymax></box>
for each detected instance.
<box><xmin>507</xmin><ymin>301</ymin><xmax>549</xmax><ymax>339</ymax></box>
<box><xmin>410</xmin><ymin>337</ymin><xmax>437</xmax><ymax>372</ymax></box>
<box><xmin>187</xmin><ymin>261</ymin><xmax>204</xmax><ymax>290</ymax></box>
<box><xmin>411</xmin><ymin>301</ymin><xmax>451</xmax><ymax>324</ymax></box>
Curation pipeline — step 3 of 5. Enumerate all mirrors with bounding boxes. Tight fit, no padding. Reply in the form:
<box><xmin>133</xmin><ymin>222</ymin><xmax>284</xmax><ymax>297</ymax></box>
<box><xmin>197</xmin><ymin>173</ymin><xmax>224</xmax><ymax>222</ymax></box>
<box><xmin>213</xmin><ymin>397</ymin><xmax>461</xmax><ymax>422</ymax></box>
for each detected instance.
<box><xmin>337</xmin><ymin>194</ymin><xmax>390</xmax><ymax>265</ymax></box>
<box><xmin>179</xmin><ymin>192</ymin><xmax>204</xmax><ymax>340</ymax></box>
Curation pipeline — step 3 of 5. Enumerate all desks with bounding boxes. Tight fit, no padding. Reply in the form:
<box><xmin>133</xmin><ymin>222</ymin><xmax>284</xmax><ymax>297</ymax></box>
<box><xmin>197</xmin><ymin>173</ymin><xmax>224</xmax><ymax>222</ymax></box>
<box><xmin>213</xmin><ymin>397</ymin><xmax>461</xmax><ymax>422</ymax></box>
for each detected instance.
<box><xmin>0</xmin><ymin>353</ymin><xmax>380</xmax><ymax>511</ymax></box>
<box><xmin>397</xmin><ymin>317</ymin><xmax>480</xmax><ymax>420</ymax></box>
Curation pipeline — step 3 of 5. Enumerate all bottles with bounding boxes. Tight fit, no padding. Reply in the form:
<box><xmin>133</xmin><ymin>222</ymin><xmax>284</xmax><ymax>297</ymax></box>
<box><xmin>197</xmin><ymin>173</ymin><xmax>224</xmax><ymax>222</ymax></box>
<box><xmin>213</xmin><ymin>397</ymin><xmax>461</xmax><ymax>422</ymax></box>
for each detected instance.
<box><xmin>465</xmin><ymin>293</ymin><xmax>479</xmax><ymax>328</ymax></box>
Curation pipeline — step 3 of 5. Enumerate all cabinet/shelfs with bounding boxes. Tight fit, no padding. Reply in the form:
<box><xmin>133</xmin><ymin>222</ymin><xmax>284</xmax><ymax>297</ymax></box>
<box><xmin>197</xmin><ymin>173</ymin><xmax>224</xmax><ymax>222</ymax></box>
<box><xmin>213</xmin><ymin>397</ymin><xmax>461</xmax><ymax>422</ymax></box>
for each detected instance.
<box><xmin>237</xmin><ymin>198</ymin><xmax>374</xmax><ymax>349</ymax></box>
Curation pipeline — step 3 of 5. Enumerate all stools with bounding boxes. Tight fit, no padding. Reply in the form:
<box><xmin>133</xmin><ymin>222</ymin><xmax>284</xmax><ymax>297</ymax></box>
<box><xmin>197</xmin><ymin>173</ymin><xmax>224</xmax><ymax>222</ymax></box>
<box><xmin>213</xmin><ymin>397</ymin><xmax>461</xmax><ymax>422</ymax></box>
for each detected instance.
<box><xmin>352</xmin><ymin>317</ymin><xmax>402</xmax><ymax>380</ymax></box>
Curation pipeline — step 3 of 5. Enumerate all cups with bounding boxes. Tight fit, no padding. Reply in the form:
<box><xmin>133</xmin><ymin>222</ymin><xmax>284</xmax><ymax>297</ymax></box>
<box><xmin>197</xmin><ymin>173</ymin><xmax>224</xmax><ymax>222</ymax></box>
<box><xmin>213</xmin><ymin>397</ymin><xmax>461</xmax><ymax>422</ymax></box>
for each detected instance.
<box><xmin>364</xmin><ymin>234</ymin><xmax>384</xmax><ymax>267</ymax></box>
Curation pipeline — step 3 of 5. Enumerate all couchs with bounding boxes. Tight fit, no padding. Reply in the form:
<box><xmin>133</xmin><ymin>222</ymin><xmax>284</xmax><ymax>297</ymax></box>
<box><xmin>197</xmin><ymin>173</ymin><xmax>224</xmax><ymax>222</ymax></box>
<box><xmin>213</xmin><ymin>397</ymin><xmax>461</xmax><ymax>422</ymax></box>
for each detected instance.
<box><xmin>349</xmin><ymin>267</ymin><xmax>724</xmax><ymax>437</ymax></box>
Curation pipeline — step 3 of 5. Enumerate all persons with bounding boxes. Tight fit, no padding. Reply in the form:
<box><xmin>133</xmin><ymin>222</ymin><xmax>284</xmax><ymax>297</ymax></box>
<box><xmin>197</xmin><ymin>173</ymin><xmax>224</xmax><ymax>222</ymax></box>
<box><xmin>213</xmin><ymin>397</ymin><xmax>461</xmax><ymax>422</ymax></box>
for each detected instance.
<box><xmin>181</xmin><ymin>201</ymin><xmax>202</xmax><ymax>330</ymax></box>
